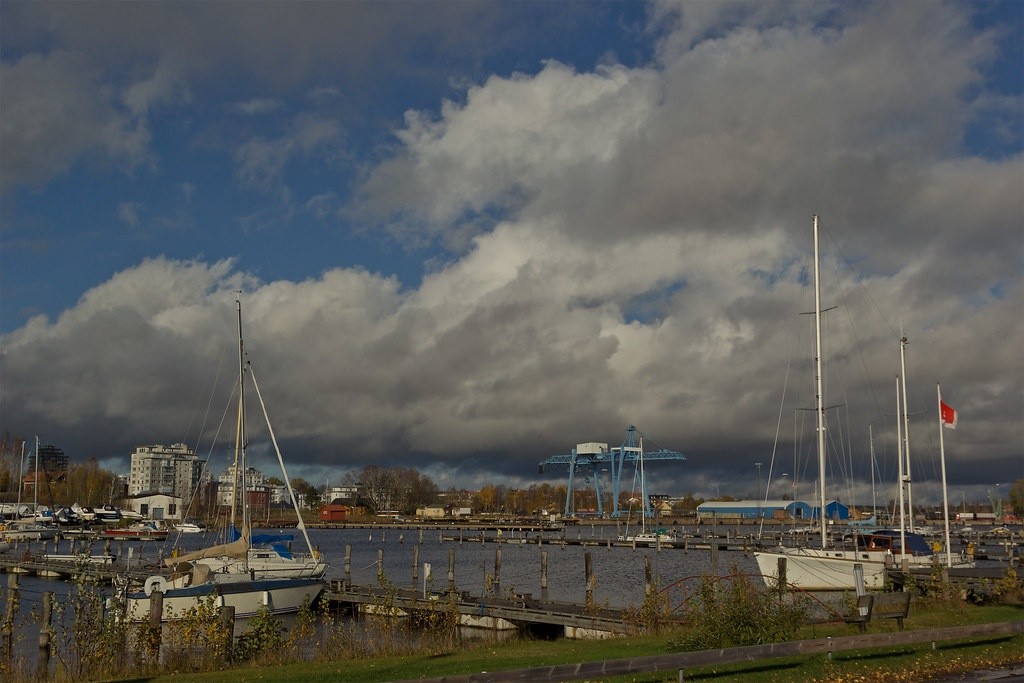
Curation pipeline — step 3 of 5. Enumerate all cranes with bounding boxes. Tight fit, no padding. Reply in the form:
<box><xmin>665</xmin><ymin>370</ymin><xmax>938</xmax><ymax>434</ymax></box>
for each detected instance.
<box><xmin>539</xmin><ymin>425</ymin><xmax>687</xmax><ymax>518</ymax></box>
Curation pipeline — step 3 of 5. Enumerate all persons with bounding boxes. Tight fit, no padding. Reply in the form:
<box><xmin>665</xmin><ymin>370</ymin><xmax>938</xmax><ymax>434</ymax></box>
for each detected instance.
<box><xmin>964</xmin><ymin>543</ymin><xmax>974</xmax><ymax>559</ymax></box>
<box><xmin>933</xmin><ymin>540</ymin><xmax>941</xmax><ymax>556</ymax></box>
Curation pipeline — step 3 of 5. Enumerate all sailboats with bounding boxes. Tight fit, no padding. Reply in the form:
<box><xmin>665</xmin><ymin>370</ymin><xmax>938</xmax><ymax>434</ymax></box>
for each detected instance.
<box><xmin>618</xmin><ymin>437</ymin><xmax>674</xmax><ymax>542</ymax></box>
<box><xmin>105</xmin><ymin>290</ymin><xmax>329</xmax><ymax>622</ymax></box>
<box><xmin>753</xmin><ymin>216</ymin><xmax>976</xmax><ymax>589</ymax></box>
<box><xmin>0</xmin><ymin>436</ymin><xmax>62</xmax><ymax>539</ymax></box>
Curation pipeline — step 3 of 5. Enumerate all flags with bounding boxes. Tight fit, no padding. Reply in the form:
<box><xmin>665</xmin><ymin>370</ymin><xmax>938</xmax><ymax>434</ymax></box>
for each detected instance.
<box><xmin>937</xmin><ymin>401</ymin><xmax>959</xmax><ymax>430</ymax></box>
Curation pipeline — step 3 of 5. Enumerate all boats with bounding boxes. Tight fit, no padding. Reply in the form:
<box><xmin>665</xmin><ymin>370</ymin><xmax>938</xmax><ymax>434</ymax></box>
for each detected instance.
<box><xmin>61</xmin><ymin>503</ymin><xmax>170</xmax><ymax>527</ymax></box>
<box><xmin>106</xmin><ymin>528</ymin><xmax>169</xmax><ymax>540</ymax></box>
<box><xmin>44</xmin><ymin>554</ymin><xmax>116</xmax><ymax>564</ymax></box>
<box><xmin>62</xmin><ymin>530</ymin><xmax>96</xmax><ymax>540</ymax></box>
<box><xmin>175</xmin><ymin>524</ymin><xmax>208</xmax><ymax>533</ymax></box>
